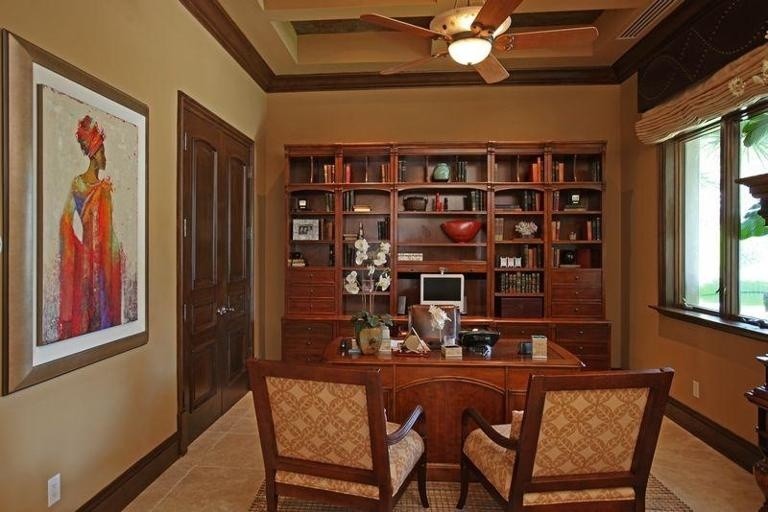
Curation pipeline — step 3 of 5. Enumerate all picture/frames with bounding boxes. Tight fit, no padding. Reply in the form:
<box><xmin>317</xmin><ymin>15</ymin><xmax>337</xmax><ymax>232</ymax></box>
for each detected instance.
<box><xmin>0</xmin><ymin>27</ymin><xmax>150</xmax><ymax>398</ymax></box>
<box><xmin>291</xmin><ymin>218</ymin><xmax>321</xmax><ymax>241</ymax></box>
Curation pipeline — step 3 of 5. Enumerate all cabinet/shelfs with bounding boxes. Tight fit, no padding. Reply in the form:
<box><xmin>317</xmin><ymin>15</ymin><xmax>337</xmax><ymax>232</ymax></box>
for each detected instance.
<box><xmin>281</xmin><ymin>139</ymin><xmax>616</xmax><ymax>370</ymax></box>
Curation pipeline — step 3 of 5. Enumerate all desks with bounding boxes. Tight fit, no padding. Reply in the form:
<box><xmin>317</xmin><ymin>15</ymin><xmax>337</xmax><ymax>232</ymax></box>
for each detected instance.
<box><xmin>318</xmin><ymin>337</ymin><xmax>587</xmax><ymax>483</ymax></box>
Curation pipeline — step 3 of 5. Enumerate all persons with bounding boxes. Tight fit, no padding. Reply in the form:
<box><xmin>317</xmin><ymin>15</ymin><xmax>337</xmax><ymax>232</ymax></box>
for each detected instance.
<box><xmin>58</xmin><ymin>114</ymin><xmax>127</xmax><ymax>341</ymax></box>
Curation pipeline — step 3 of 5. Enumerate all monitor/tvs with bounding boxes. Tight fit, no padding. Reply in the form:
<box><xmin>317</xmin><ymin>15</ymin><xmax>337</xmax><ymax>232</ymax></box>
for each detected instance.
<box><xmin>419</xmin><ymin>273</ymin><xmax>465</xmax><ymax>305</ymax></box>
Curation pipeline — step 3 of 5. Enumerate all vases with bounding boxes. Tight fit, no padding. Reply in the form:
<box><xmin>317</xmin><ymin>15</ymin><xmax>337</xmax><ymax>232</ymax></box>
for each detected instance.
<box><xmin>357</xmin><ymin>327</ymin><xmax>383</xmax><ymax>356</ymax></box>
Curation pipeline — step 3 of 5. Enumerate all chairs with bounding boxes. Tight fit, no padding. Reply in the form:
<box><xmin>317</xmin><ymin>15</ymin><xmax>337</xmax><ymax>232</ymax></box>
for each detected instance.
<box><xmin>456</xmin><ymin>367</ymin><xmax>674</xmax><ymax>512</ymax></box>
<box><xmin>407</xmin><ymin>303</ymin><xmax>461</xmax><ymax>348</ymax></box>
<box><xmin>245</xmin><ymin>356</ymin><xmax>429</xmax><ymax>512</ymax></box>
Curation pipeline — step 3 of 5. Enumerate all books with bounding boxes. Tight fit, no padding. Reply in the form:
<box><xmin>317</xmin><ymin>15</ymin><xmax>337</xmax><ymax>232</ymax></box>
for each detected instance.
<box><xmin>582</xmin><ymin>220</ymin><xmax>592</xmax><ymax>240</ymax></box>
<box><xmin>596</xmin><ymin>217</ymin><xmax>601</xmax><ymax>240</ymax></box>
<box><xmin>319</xmin><ymin>217</ymin><xmax>390</xmax><ymax>267</ymax></box>
<box><xmin>499</xmin><ymin>218</ymin><xmax>504</xmax><ymax>241</ymax></box>
<box><xmin>495</xmin><ymin>218</ymin><xmax>499</xmax><ymax>241</ymax></box>
<box><xmin>291</xmin><ymin>262</ymin><xmax>306</xmax><ymax>267</ymax></box>
<box><xmin>500</xmin><ymin>245</ymin><xmax>542</xmax><ymax>294</ymax></box>
<box><xmin>436</xmin><ymin>192</ymin><xmax>440</xmax><ymax>210</ymax></box>
<box><xmin>556</xmin><ymin>220</ymin><xmax>560</xmax><ymax>240</ymax></box>
<box><xmin>551</xmin><ymin>247</ymin><xmax>582</xmax><ymax>268</ymax></box>
<box><xmin>590</xmin><ymin>218</ymin><xmax>596</xmax><ymax>240</ymax></box>
<box><xmin>323</xmin><ymin>164</ymin><xmax>372</xmax><ymax>213</ymax></box>
<box><xmin>439</xmin><ymin>202</ymin><xmax>443</xmax><ymax>211</ymax></box>
<box><xmin>292</xmin><ymin>258</ymin><xmax>305</xmax><ymax>263</ymax></box>
<box><xmin>380</xmin><ymin>160</ymin><xmax>408</xmax><ymax>182</ymax></box>
<box><xmin>456</xmin><ymin>160</ymin><xmax>484</xmax><ymax>211</ymax></box>
<box><xmin>495</xmin><ymin>157</ymin><xmax>565</xmax><ymax>211</ymax></box>
<box><xmin>396</xmin><ymin>252</ymin><xmax>424</xmax><ymax>262</ymax></box>
<box><xmin>551</xmin><ymin>221</ymin><xmax>556</xmax><ymax>241</ymax></box>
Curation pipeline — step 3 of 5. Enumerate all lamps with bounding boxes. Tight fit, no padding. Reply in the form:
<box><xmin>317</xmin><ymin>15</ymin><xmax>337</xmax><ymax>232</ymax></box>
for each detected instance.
<box><xmin>447</xmin><ymin>35</ymin><xmax>493</xmax><ymax>66</ymax></box>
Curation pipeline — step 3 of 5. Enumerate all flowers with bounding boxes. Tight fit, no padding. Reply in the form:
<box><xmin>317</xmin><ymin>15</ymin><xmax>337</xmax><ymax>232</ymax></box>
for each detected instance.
<box><xmin>344</xmin><ymin>237</ymin><xmax>394</xmax><ymax>334</ymax></box>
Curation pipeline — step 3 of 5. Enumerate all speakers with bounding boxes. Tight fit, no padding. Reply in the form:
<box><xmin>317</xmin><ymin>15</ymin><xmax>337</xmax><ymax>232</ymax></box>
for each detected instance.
<box><xmin>459</xmin><ymin>295</ymin><xmax>468</xmax><ymax>315</ymax></box>
<box><xmin>397</xmin><ymin>295</ymin><xmax>407</xmax><ymax>314</ymax></box>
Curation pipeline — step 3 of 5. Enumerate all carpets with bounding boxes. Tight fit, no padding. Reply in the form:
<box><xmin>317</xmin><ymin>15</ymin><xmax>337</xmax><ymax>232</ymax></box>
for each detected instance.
<box><xmin>248</xmin><ymin>472</ymin><xmax>695</xmax><ymax>512</ymax></box>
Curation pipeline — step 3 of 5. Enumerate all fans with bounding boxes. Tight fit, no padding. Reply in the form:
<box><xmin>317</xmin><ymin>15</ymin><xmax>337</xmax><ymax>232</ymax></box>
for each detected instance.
<box><xmin>359</xmin><ymin>0</ymin><xmax>600</xmax><ymax>85</ymax></box>
